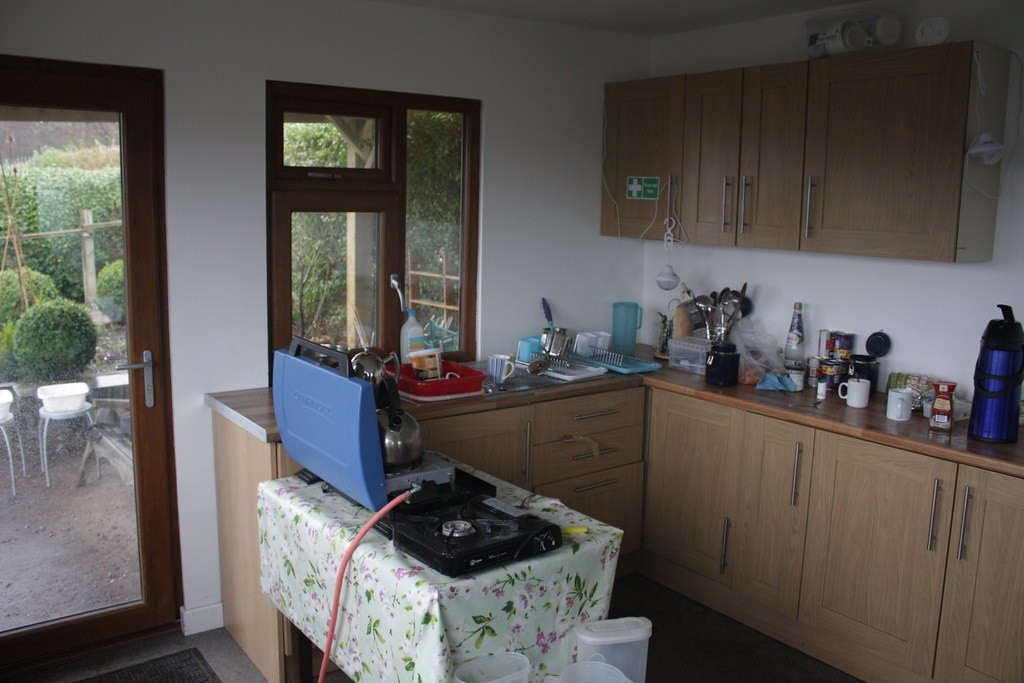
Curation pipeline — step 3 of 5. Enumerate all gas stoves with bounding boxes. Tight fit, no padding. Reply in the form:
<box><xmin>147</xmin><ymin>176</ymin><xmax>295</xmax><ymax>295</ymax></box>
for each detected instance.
<box><xmin>298</xmin><ymin>453</ymin><xmax>566</xmax><ymax>577</ymax></box>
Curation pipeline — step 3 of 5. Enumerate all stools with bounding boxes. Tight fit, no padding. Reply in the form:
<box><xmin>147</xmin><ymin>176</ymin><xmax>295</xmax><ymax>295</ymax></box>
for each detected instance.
<box><xmin>0</xmin><ymin>413</ymin><xmax>26</xmax><ymax>499</ymax></box>
<box><xmin>38</xmin><ymin>402</ymin><xmax>100</xmax><ymax>487</ymax></box>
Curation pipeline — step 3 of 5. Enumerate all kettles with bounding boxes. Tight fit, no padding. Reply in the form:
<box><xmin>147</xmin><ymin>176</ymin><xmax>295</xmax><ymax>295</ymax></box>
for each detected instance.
<box><xmin>375</xmin><ymin>376</ymin><xmax>424</xmax><ymax>478</ymax></box>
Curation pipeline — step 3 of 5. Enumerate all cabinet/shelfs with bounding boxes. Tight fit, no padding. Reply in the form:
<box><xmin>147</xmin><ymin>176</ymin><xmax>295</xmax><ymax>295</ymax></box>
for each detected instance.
<box><xmin>601</xmin><ymin>39</ymin><xmax>1011</xmax><ymax>263</ymax></box>
<box><xmin>230</xmin><ymin>340</ymin><xmax>1024</xmax><ymax>682</ymax></box>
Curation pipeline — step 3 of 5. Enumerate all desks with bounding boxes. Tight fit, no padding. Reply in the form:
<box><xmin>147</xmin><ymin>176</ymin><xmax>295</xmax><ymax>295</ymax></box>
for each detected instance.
<box><xmin>257</xmin><ymin>451</ymin><xmax>624</xmax><ymax>682</ymax></box>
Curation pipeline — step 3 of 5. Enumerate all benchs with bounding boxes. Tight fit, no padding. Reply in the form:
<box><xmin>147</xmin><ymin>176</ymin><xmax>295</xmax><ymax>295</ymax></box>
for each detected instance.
<box><xmin>74</xmin><ymin>399</ymin><xmax>134</xmax><ymax>488</ymax></box>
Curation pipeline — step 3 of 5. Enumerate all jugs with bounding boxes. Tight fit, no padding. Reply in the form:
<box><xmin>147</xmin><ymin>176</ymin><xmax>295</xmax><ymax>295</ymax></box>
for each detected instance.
<box><xmin>612</xmin><ymin>302</ymin><xmax>643</xmax><ymax>357</ymax></box>
<box><xmin>967</xmin><ymin>304</ymin><xmax>1024</xmax><ymax>446</ymax></box>
<box><xmin>349</xmin><ymin>347</ymin><xmax>400</xmax><ymax>394</ymax></box>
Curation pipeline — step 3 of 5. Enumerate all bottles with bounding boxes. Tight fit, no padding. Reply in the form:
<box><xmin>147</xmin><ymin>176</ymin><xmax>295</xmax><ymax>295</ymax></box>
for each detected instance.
<box><xmin>399</xmin><ymin>308</ymin><xmax>425</xmax><ymax>363</ymax></box>
<box><xmin>850</xmin><ymin>332</ymin><xmax>890</xmax><ymax>394</ymax></box>
<box><xmin>784</xmin><ymin>302</ymin><xmax>806</xmax><ymax>371</ymax></box>
<box><xmin>704</xmin><ymin>341</ymin><xmax>740</xmax><ymax>388</ymax></box>
<box><xmin>927</xmin><ymin>381</ymin><xmax>957</xmax><ymax>432</ymax></box>
<box><xmin>816</xmin><ymin>379</ymin><xmax>830</xmax><ymax>400</ymax></box>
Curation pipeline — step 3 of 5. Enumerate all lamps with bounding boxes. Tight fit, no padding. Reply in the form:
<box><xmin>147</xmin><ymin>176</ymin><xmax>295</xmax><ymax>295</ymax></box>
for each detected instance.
<box><xmin>969</xmin><ymin>133</ymin><xmax>1005</xmax><ymax>166</ymax></box>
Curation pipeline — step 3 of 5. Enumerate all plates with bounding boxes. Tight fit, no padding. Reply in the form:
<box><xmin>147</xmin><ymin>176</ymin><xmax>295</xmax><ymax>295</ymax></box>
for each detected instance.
<box><xmin>916</xmin><ymin>17</ymin><xmax>949</xmax><ymax>45</ymax></box>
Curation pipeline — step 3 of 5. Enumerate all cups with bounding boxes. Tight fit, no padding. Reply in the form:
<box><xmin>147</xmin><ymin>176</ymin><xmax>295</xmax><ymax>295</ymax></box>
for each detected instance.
<box><xmin>837</xmin><ymin>378</ymin><xmax>871</xmax><ymax>409</ymax></box>
<box><xmin>519</xmin><ymin>337</ymin><xmax>540</xmax><ymax>364</ymax></box>
<box><xmin>409</xmin><ymin>347</ymin><xmax>445</xmax><ymax>381</ymax></box>
<box><xmin>886</xmin><ymin>388</ymin><xmax>913</xmax><ymax>421</ymax></box>
<box><xmin>812</xmin><ymin>13</ymin><xmax>899</xmax><ymax>53</ymax></box>
<box><xmin>488</xmin><ymin>354</ymin><xmax>515</xmax><ymax>384</ymax></box>
<box><xmin>576</xmin><ymin>331</ymin><xmax>611</xmax><ymax>356</ymax></box>
<box><xmin>544</xmin><ymin>326</ymin><xmax>567</xmax><ymax>351</ymax></box>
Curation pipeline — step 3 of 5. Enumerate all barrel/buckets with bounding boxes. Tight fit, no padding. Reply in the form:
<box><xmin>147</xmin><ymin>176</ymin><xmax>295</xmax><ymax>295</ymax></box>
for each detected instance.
<box><xmin>455</xmin><ymin>616</ymin><xmax>653</xmax><ymax>683</ymax></box>
